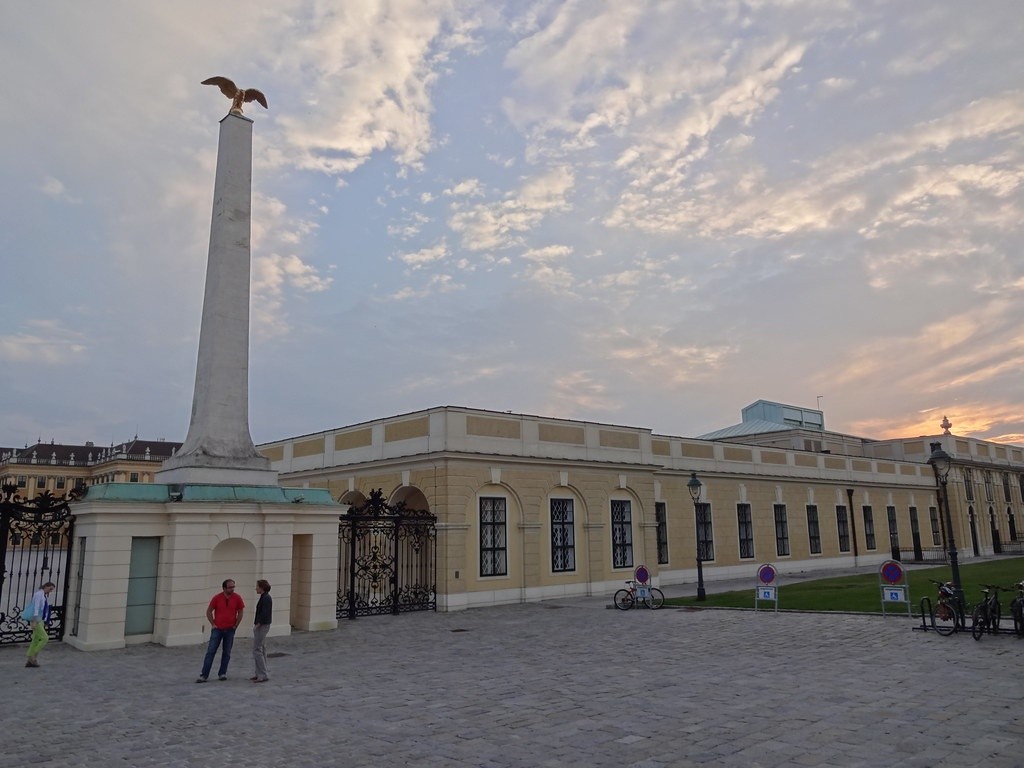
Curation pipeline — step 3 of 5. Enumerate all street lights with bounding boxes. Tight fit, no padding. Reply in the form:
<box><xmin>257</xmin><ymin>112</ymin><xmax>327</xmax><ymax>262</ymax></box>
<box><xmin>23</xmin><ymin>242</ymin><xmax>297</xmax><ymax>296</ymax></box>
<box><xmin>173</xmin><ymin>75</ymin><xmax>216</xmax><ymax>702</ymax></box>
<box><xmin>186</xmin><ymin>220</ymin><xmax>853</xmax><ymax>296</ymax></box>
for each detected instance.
<box><xmin>926</xmin><ymin>440</ymin><xmax>967</xmax><ymax>608</ymax></box>
<box><xmin>686</xmin><ymin>472</ymin><xmax>706</xmax><ymax>600</ymax></box>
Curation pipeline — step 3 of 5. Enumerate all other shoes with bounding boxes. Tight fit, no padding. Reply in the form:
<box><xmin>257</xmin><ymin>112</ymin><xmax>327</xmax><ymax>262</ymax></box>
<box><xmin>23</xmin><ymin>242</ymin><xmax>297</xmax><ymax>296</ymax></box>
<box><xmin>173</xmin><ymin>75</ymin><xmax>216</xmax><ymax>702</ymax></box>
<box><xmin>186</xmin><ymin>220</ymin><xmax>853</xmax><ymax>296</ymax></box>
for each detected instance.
<box><xmin>250</xmin><ymin>675</ymin><xmax>257</xmax><ymax>680</ymax></box>
<box><xmin>254</xmin><ymin>675</ymin><xmax>268</xmax><ymax>682</ymax></box>
<box><xmin>25</xmin><ymin>659</ymin><xmax>41</xmax><ymax>667</ymax></box>
<box><xmin>196</xmin><ymin>675</ymin><xmax>206</xmax><ymax>683</ymax></box>
<box><xmin>219</xmin><ymin>674</ymin><xmax>227</xmax><ymax>680</ymax></box>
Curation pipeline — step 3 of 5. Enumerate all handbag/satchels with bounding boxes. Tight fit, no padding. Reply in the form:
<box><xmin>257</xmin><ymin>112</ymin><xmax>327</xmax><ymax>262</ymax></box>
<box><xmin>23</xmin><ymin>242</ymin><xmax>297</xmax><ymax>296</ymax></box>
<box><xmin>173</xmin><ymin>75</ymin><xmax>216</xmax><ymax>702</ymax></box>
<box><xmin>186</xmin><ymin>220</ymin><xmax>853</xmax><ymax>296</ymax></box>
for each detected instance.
<box><xmin>22</xmin><ymin>592</ymin><xmax>42</xmax><ymax>623</ymax></box>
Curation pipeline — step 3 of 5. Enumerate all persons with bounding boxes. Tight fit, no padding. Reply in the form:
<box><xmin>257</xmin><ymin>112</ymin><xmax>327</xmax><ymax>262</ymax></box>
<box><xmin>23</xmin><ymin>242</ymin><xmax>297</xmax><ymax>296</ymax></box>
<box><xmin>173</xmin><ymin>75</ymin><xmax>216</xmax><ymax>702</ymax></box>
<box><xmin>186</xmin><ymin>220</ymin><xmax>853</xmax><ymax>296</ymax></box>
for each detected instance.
<box><xmin>20</xmin><ymin>582</ymin><xmax>56</xmax><ymax>667</ymax></box>
<box><xmin>196</xmin><ymin>579</ymin><xmax>245</xmax><ymax>683</ymax></box>
<box><xmin>250</xmin><ymin>580</ymin><xmax>273</xmax><ymax>683</ymax></box>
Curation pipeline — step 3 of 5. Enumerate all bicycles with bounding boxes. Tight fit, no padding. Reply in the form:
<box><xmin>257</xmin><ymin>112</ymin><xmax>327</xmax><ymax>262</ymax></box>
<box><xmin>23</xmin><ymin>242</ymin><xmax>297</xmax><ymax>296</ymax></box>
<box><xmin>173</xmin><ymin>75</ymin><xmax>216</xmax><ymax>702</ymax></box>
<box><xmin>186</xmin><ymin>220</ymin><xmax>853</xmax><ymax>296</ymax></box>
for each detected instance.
<box><xmin>929</xmin><ymin>578</ymin><xmax>960</xmax><ymax>636</ymax></box>
<box><xmin>971</xmin><ymin>583</ymin><xmax>1010</xmax><ymax>641</ymax></box>
<box><xmin>614</xmin><ymin>581</ymin><xmax>664</xmax><ymax>610</ymax></box>
<box><xmin>1005</xmin><ymin>582</ymin><xmax>1024</xmax><ymax>639</ymax></box>
